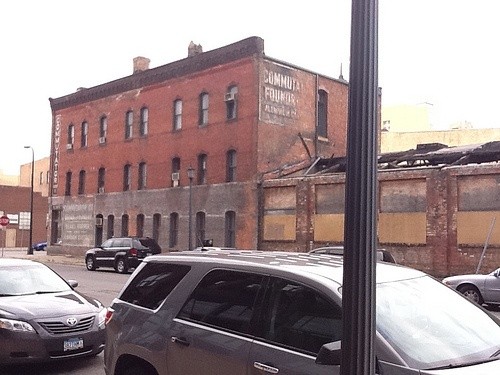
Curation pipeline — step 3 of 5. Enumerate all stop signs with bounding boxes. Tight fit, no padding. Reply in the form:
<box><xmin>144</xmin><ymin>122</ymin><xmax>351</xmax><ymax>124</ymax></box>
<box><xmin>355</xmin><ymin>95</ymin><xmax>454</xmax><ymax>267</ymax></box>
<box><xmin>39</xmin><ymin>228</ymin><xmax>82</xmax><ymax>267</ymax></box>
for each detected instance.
<box><xmin>0</xmin><ymin>216</ymin><xmax>10</xmax><ymax>227</ymax></box>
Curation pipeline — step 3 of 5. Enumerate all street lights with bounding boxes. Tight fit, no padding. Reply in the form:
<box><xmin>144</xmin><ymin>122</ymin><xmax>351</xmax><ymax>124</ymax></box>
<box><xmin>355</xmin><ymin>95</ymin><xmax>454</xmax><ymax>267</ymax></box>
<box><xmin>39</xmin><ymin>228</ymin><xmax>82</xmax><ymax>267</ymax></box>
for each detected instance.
<box><xmin>23</xmin><ymin>145</ymin><xmax>33</xmax><ymax>254</ymax></box>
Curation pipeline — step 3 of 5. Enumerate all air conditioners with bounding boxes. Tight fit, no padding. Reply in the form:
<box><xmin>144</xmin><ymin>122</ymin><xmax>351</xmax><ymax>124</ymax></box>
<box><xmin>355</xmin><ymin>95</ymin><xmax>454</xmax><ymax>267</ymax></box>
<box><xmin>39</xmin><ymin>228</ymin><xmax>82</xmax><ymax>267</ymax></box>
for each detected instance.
<box><xmin>224</xmin><ymin>92</ymin><xmax>237</xmax><ymax>101</ymax></box>
<box><xmin>172</xmin><ymin>173</ymin><xmax>180</xmax><ymax>180</ymax></box>
<box><xmin>95</xmin><ymin>218</ymin><xmax>103</xmax><ymax>225</ymax></box>
<box><xmin>99</xmin><ymin>137</ymin><xmax>106</xmax><ymax>143</ymax></box>
<box><xmin>99</xmin><ymin>188</ymin><xmax>105</xmax><ymax>193</ymax></box>
<box><xmin>67</xmin><ymin>144</ymin><xmax>73</xmax><ymax>149</ymax></box>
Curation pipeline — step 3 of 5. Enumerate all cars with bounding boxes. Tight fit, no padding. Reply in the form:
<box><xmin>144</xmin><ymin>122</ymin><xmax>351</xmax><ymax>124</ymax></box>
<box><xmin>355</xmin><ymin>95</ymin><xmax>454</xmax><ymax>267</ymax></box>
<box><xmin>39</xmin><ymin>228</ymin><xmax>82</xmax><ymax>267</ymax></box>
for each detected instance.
<box><xmin>0</xmin><ymin>257</ymin><xmax>107</xmax><ymax>375</ymax></box>
<box><xmin>441</xmin><ymin>267</ymin><xmax>500</xmax><ymax>306</ymax></box>
<box><xmin>34</xmin><ymin>241</ymin><xmax>48</xmax><ymax>251</ymax></box>
<box><xmin>306</xmin><ymin>246</ymin><xmax>396</xmax><ymax>264</ymax></box>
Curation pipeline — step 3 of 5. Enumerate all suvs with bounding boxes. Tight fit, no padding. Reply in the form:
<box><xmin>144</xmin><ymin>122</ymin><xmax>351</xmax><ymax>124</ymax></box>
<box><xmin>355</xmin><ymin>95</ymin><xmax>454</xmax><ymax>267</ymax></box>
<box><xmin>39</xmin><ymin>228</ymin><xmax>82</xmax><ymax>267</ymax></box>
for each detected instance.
<box><xmin>101</xmin><ymin>244</ymin><xmax>500</xmax><ymax>375</ymax></box>
<box><xmin>84</xmin><ymin>235</ymin><xmax>162</xmax><ymax>274</ymax></box>
